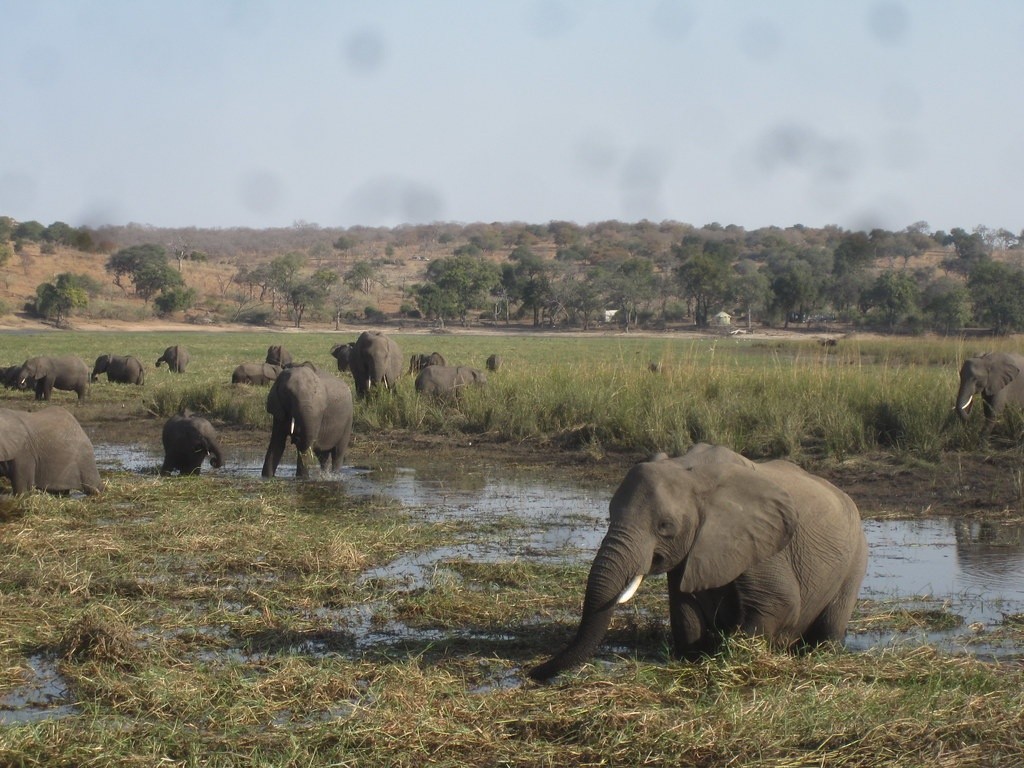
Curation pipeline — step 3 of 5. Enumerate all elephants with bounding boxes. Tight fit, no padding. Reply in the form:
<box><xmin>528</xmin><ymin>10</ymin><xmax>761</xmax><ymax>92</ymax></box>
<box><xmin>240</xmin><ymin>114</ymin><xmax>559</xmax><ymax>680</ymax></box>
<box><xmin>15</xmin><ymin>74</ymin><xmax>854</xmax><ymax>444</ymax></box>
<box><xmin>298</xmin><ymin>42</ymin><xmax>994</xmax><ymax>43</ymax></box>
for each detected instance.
<box><xmin>528</xmin><ymin>441</ymin><xmax>869</xmax><ymax>689</ymax></box>
<box><xmin>0</xmin><ymin>330</ymin><xmax>504</xmax><ymax>499</ymax></box>
<box><xmin>951</xmin><ymin>351</ymin><xmax>1024</xmax><ymax>442</ymax></box>
